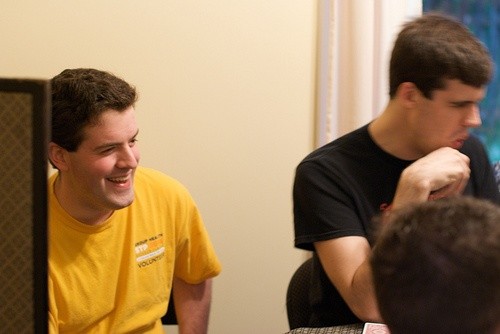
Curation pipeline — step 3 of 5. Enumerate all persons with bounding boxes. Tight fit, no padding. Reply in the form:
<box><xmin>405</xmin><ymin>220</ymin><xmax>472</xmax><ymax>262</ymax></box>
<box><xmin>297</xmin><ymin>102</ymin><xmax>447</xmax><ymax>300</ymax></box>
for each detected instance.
<box><xmin>48</xmin><ymin>68</ymin><xmax>222</xmax><ymax>334</ymax></box>
<box><xmin>294</xmin><ymin>13</ymin><xmax>500</xmax><ymax>334</ymax></box>
